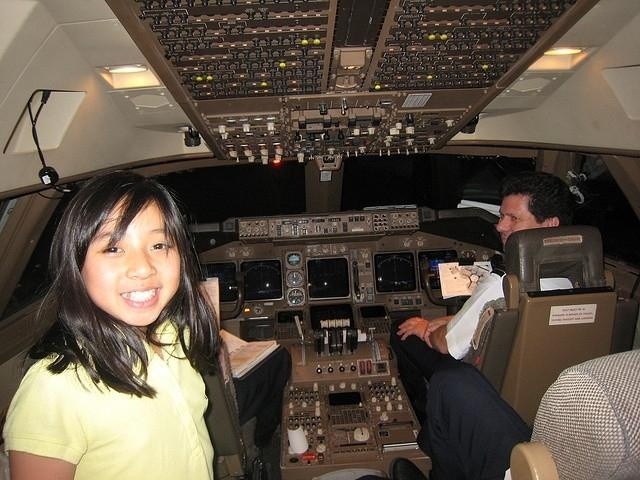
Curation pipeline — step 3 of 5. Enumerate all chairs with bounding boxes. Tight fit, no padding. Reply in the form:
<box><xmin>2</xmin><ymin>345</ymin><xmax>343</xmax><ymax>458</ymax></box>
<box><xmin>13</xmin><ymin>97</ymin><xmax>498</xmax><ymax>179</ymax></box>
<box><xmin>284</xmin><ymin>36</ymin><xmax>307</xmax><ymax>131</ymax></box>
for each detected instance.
<box><xmin>182</xmin><ymin>314</ymin><xmax>283</xmax><ymax>480</ymax></box>
<box><xmin>502</xmin><ymin>441</ymin><xmax>560</xmax><ymax>480</ymax></box>
<box><xmin>415</xmin><ymin>225</ymin><xmax>639</xmax><ymax>479</ymax></box>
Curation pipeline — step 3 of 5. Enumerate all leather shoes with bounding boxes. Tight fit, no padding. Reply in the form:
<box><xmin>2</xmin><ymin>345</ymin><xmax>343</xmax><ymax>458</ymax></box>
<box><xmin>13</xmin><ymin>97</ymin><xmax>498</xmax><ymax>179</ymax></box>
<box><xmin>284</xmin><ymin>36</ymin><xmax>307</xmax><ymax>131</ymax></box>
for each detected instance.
<box><xmin>390</xmin><ymin>457</ymin><xmax>427</xmax><ymax>480</ymax></box>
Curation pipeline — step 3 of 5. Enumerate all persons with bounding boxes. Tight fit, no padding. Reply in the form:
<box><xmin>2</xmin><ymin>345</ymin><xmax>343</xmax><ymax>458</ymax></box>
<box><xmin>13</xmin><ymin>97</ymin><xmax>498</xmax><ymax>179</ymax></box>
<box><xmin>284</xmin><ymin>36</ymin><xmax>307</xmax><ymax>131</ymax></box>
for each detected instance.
<box><xmin>391</xmin><ymin>170</ymin><xmax>640</xmax><ymax>479</ymax></box>
<box><xmin>222</xmin><ymin>329</ymin><xmax>293</xmax><ymax>449</ymax></box>
<box><xmin>2</xmin><ymin>166</ymin><xmax>221</xmax><ymax>479</ymax></box>
<box><xmin>392</xmin><ymin>358</ymin><xmax>534</xmax><ymax>480</ymax></box>
